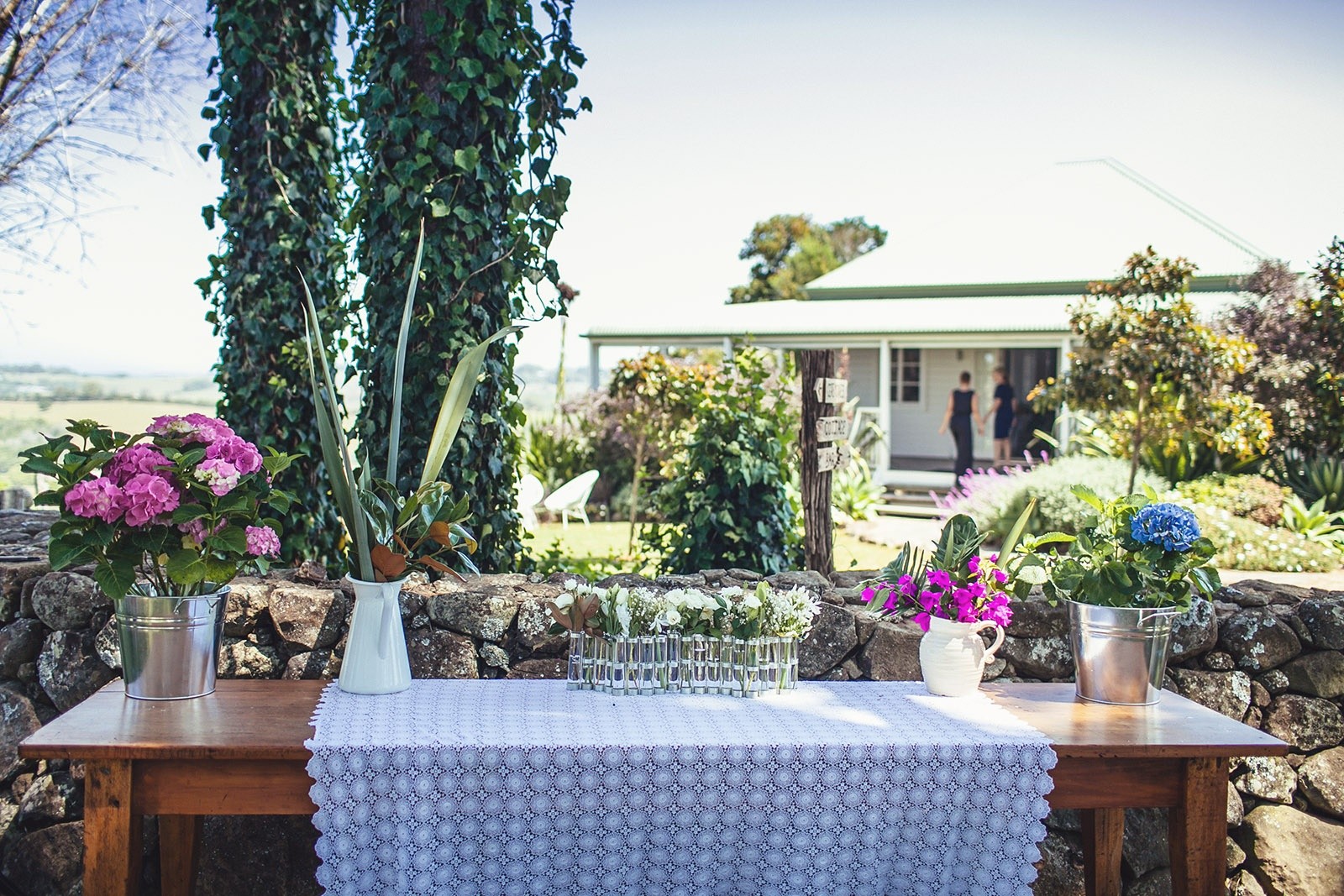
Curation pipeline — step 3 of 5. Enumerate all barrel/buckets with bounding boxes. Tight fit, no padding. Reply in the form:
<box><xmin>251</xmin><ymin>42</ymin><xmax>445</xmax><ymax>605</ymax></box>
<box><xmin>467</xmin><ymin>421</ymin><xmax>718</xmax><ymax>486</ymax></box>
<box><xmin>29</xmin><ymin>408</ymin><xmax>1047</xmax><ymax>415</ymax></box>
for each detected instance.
<box><xmin>115</xmin><ymin>580</ymin><xmax>232</xmax><ymax>701</ymax></box>
<box><xmin>1065</xmin><ymin>598</ymin><xmax>1181</xmax><ymax>704</ymax></box>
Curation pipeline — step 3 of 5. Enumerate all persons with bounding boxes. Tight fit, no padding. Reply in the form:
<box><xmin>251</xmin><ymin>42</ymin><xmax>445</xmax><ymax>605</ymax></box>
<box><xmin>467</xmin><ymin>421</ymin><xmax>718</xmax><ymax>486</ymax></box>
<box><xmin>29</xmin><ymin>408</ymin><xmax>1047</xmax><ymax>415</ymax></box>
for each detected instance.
<box><xmin>940</xmin><ymin>372</ymin><xmax>986</xmax><ymax>487</ymax></box>
<box><xmin>983</xmin><ymin>364</ymin><xmax>1018</xmax><ymax>471</ymax></box>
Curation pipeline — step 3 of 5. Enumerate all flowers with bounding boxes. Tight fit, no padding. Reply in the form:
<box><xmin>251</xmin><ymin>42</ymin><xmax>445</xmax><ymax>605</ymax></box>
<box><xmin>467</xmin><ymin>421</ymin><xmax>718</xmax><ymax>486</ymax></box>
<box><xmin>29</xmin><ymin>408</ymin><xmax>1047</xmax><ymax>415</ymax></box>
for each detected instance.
<box><xmin>547</xmin><ymin>575</ymin><xmax>823</xmax><ymax>692</ymax></box>
<box><xmin>853</xmin><ymin>499</ymin><xmax>1221</xmax><ymax>634</ymax></box>
<box><xmin>18</xmin><ymin>410</ymin><xmax>306</xmax><ymax>601</ymax></box>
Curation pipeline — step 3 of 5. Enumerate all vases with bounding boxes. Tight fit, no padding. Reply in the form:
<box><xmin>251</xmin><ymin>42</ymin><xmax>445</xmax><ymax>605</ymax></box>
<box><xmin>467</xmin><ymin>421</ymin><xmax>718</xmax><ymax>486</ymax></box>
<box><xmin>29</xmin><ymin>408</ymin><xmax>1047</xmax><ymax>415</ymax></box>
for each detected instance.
<box><xmin>338</xmin><ymin>572</ymin><xmax>412</xmax><ymax>694</ymax></box>
<box><xmin>115</xmin><ymin>580</ymin><xmax>232</xmax><ymax>700</ymax></box>
<box><xmin>1064</xmin><ymin>598</ymin><xmax>1181</xmax><ymax>705</ymax></box>
<box><xmin>919</xmin><ymin>616</ymin><xmax>1005</xmax><ymax>697</ymax></box>
<box><xmin>566</xmin><ymin>633</ymin><xmax>799</xmax><ymax>698</ymax></box>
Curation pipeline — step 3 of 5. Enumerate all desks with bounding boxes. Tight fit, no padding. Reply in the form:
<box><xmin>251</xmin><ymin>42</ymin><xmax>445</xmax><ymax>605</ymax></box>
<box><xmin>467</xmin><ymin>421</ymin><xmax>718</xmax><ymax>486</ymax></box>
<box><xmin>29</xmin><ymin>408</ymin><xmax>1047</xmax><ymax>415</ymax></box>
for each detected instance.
<box><xmin>18</xmin><ymin>680</ymin><xmax>1292</xmax><ymax>896</ymax></box>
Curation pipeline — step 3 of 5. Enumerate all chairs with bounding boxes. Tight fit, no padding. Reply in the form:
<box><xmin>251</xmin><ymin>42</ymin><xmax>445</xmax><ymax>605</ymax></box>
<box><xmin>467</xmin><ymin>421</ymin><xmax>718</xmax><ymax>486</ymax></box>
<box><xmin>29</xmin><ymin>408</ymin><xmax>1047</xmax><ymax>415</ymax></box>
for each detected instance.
<box><xmin>519</xmin><ymin>470</ymin><xmax>600</xmax><ymax>534</ymax></box>
